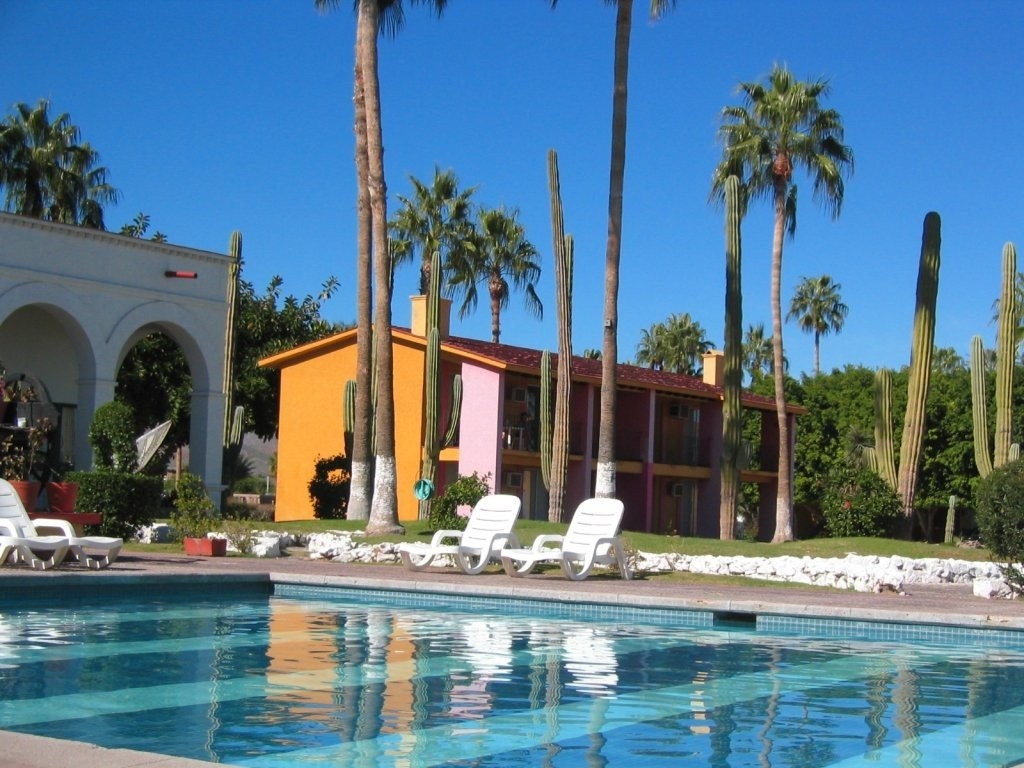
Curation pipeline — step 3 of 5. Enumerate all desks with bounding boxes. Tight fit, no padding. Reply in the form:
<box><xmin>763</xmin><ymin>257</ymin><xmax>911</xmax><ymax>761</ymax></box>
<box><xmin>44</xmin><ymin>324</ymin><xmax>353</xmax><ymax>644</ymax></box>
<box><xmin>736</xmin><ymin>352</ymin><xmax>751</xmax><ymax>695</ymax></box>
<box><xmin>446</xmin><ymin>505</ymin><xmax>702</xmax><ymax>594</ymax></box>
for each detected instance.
<box><xmin>503</xmin><ymin>425</ymin><xmax>524</xmax><ymax>450</ymax></box>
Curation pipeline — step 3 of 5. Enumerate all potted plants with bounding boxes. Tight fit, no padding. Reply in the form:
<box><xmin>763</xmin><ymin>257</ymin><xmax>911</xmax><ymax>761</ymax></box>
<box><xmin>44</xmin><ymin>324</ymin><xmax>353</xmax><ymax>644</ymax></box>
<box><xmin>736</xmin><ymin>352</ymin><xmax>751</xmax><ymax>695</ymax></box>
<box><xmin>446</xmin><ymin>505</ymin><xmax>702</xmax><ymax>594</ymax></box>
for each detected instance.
<box><xmin>0</xmin><ymin>417</ymin><xmax>79</xmax><ymax>513</ymax></box>
<box><xmin>166</xmin><ymin>471</ymin><xmax>227</xmax><ymax>556</ymax></box>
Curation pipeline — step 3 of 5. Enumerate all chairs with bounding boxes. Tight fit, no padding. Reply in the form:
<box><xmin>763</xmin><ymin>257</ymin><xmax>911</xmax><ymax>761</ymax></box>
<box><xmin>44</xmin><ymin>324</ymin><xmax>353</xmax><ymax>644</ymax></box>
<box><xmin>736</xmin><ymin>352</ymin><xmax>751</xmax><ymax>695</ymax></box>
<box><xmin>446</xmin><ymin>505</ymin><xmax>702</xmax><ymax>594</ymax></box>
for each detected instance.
<box><xmin>399</xmin><ymin>495</ymin><xmax>525</xmax><ymax>575</ymax></box>
<box><xmin>501</xmin><ymin>497</ymin><xmax>634</xmax><ymax>581</ymax></box>
<box><xmin>0</xmin><ymin>478</ymin><xmax>122</xmax><ymax>568</ymax></box>
